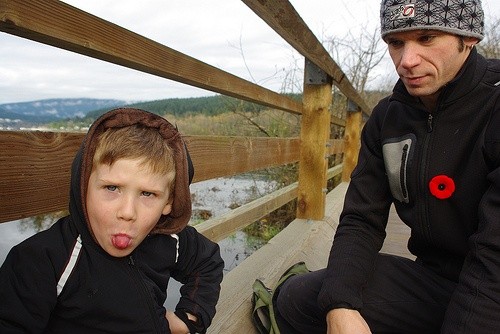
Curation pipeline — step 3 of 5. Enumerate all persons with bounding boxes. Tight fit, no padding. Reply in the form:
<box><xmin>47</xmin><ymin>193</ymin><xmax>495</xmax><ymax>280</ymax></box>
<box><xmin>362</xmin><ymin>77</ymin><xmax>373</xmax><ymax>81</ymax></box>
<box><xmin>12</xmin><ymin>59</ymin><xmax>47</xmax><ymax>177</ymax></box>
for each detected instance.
<box><xmin>1</xmin><ymin>107</ymin><xmax>225</xmax><ymax>334</ymax></box>
<box><xmin>275</xmin><ymin>0</ymin><xmax>500</xmax><ymax>334</ymax></box>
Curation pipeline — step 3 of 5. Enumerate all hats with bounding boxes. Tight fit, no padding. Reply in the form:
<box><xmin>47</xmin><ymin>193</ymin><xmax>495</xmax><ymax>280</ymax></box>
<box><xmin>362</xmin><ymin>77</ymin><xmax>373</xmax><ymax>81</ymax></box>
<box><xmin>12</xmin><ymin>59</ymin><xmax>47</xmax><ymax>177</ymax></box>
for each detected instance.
<box><xmin>381</xmin><ymin>0</ymin><xmax>484</xmax><ymax>43</ymax></box>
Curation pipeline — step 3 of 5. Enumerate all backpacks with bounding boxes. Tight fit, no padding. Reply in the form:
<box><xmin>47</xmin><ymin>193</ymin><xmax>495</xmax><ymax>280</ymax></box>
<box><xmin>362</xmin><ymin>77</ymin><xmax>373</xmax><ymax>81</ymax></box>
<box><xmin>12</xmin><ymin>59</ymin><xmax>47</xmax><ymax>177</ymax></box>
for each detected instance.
<box><xmin>250</xmin><ymin>261</ymin><xmax>314</xmax><ymax>334</ymax></box>
